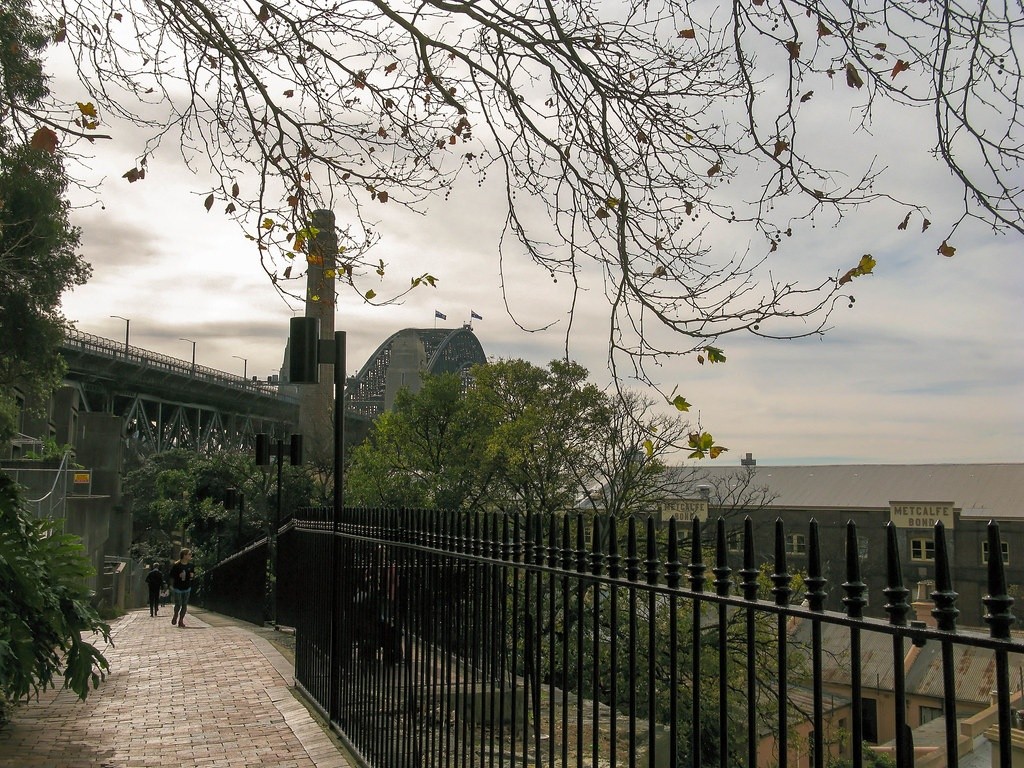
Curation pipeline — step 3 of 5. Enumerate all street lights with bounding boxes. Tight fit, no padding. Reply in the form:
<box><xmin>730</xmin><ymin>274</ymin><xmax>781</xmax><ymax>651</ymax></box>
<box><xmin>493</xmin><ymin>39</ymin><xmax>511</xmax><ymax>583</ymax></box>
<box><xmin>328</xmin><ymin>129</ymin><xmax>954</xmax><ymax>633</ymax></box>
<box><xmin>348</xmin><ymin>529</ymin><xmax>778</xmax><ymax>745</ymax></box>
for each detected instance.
<box><xmin>110</xmin><ymin>315</ymin><xmax>131</xmax><ymax>358</ymax></box>
<box><xmin>179</xmin><ymin>337</ymin><xmax>196</xmax><ymax>370</ymax></box>
<box><xmin>233</xmin><ymin>355</ymin><xmax>249</xmax><ymax>388</ymax></box>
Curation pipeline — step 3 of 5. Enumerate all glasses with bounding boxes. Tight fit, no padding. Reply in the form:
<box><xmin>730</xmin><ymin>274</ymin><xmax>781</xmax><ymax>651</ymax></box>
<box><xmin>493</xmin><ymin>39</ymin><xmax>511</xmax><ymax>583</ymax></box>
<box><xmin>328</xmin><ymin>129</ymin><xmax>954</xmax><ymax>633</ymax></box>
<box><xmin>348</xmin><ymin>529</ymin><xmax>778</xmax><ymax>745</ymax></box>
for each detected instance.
<box><xmin>188</xmin><ymin>553</ymin><xmax>192</xmax><ymax>555</ymax></box>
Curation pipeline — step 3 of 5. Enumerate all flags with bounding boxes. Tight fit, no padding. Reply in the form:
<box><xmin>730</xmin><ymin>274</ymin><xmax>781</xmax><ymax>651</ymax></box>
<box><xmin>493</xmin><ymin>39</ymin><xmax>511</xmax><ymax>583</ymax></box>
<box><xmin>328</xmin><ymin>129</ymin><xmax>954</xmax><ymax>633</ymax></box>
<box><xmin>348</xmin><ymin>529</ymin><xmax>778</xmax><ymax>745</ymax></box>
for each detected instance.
<box><xmin>436</xmin><ymin>311</ymin><xmax>446</xmax><ymax>320</ymax></box>
<box><xmin>472</xmin><ymin>310</ymin><xmax>483</xmax><ymax>320</ymax></box>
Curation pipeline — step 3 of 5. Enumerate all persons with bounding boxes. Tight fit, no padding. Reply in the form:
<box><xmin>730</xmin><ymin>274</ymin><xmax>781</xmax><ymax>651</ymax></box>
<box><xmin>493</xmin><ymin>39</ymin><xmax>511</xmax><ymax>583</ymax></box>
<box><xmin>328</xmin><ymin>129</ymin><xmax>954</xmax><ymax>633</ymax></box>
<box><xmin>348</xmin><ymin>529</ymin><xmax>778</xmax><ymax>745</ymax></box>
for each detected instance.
<box><xmin>159</xmin><ymin>581</ymin><xmax>169</xmax><ymax>607</ymax></box>
<box><xmin>167</xmin><ymin>549</ymin><xmax>195</xmax><ymax>627</ymax></box>
<box><xmin>145</xmin><ymin>562</ymin><xmax>165</xmax><ymax>617</ymax></box>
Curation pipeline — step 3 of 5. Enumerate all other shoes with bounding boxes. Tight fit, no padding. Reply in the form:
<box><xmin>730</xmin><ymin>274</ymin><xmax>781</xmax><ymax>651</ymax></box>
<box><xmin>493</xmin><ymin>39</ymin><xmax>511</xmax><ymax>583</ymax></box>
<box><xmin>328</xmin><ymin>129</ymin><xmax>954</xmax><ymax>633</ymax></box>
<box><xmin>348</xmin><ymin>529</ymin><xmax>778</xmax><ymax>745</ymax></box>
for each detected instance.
<box><xmin>172</xmin><ymin>615</ymin><xmax>178</xmax><ymax>624</ymax></box>
<box><xmin>179</xmin><ymin>622</ymin><xmax>185</xmax><ymax>627</ymax></box>
